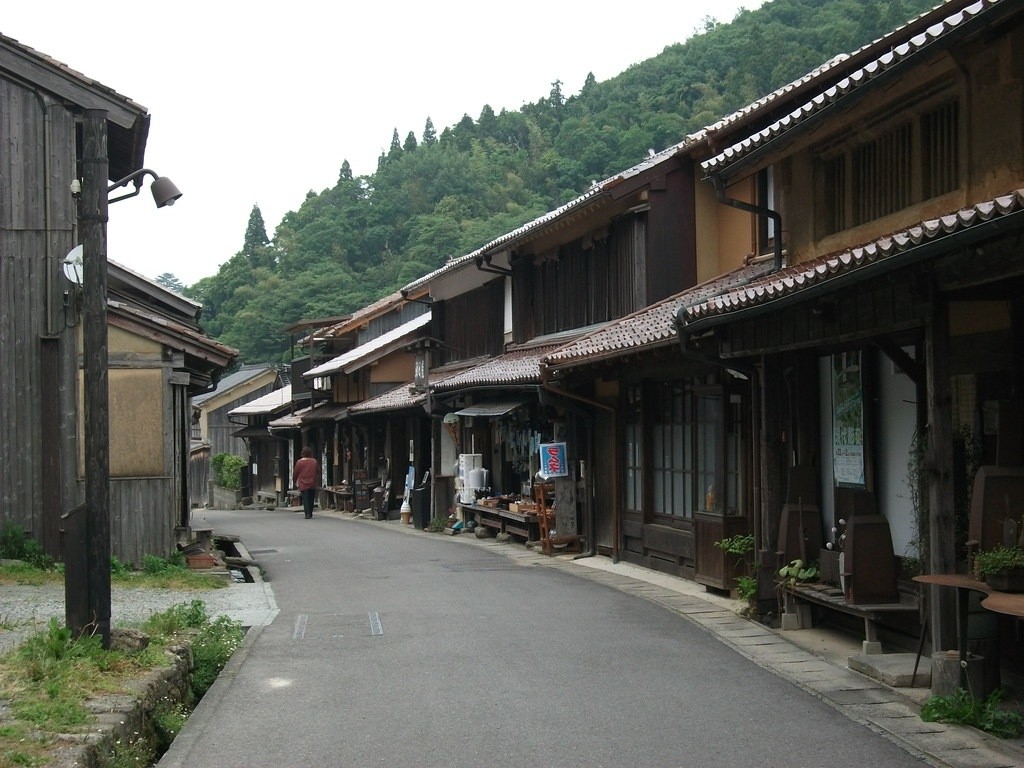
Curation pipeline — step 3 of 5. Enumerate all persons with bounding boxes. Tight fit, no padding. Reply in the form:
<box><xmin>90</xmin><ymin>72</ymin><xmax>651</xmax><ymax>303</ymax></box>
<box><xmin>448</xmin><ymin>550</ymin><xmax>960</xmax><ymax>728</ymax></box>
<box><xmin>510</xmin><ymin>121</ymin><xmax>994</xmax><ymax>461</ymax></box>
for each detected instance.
<box><xmin>291</xmin><ymin>446</ymin><xmax>322</xmax><ymax>520</ymax></box>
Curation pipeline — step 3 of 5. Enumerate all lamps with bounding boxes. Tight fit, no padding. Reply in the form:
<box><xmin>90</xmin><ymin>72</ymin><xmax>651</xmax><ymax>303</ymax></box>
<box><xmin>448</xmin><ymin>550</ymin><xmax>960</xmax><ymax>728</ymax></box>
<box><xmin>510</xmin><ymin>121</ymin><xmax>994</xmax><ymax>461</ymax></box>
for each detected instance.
<box><xmin>107</xmin><ymin>169</ymin><xmax>183</xmax><ymax>209</ymax></box>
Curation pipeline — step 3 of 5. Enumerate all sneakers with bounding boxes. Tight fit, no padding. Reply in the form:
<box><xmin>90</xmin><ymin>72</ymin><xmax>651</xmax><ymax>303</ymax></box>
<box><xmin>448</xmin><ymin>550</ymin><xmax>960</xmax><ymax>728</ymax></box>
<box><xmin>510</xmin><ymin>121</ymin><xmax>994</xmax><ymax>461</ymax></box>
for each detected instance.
<box><xmin>304</xmin><ymin>514</ymin><xmax>312</xmax><ymax>519</ymax></box>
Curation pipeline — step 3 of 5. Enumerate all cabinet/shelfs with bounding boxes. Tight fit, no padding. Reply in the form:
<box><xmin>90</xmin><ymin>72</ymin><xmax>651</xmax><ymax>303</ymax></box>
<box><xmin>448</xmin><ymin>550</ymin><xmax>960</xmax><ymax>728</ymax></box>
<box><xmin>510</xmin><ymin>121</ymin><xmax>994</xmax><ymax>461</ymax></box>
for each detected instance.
<box><xmin>535</xmin><ymin>482</ymin><xmax>582</xmax><ymax>556</ymax></box>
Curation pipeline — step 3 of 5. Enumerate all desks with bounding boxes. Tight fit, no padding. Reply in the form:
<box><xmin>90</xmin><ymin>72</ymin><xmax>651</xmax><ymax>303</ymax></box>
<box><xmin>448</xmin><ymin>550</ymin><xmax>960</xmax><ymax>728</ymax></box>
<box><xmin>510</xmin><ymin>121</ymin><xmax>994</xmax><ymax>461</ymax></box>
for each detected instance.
<box><xmin>912</xmin><ymin>574</ymin><xmax>1024</xmax><ymax>701</ymax></box>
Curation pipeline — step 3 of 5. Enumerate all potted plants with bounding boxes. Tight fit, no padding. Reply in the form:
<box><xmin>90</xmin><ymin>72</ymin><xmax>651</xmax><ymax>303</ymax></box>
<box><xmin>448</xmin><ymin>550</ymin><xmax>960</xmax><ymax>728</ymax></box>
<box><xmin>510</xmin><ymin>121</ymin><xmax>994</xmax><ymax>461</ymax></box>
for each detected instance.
<box><xmin>985</xmin><ymin>545</ymin><xmax>1023</xmax><ymax>593</ymax></box>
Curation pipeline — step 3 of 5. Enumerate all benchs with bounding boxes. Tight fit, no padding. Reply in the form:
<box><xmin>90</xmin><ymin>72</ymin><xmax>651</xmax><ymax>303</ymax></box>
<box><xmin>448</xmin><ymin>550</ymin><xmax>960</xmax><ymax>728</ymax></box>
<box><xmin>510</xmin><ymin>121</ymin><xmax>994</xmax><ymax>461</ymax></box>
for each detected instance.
<box><xmin>782</xmin><ymin>548</ymin><xmax>924</xmax><ymax>655</ymax></box>
